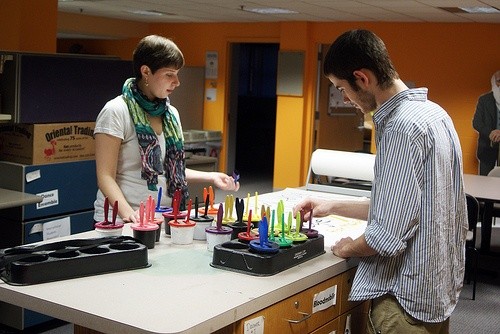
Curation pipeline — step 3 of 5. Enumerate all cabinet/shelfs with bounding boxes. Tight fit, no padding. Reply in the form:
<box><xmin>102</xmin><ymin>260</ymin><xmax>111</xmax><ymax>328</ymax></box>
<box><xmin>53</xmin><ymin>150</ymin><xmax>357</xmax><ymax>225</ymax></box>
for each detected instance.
<box><xmin>0</xmin><ymin>188</ymin><xmax>372</xmax><ymax>334</ymax></box>
<box><xmin>0</xmin><ymin>161</ymin><xmax>99</xmax><ymax>331</ymax></box>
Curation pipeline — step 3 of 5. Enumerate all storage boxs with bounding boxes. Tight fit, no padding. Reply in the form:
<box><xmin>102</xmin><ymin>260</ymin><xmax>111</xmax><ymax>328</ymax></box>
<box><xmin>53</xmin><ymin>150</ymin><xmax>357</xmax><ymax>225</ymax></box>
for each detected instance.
<box><xmin>0</xmin><ymin>122</ymin><xmax>96</xmax><ymax>165</ymax></box>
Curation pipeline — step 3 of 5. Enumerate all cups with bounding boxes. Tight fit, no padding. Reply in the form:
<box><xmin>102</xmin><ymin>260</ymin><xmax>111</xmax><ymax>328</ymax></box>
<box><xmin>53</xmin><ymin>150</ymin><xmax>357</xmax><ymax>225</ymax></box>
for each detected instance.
<box><xmin>206</xmin><ymin>226</ymin><xmax>233</xmax><ymax>252</ymax></box>
<box><xmin>155</xmin><ymin>208</ymin><xmax>188</xmax><ymax>238</ymax></box>
<box><xmin>95</xmin><ymin>221</ymin><xmax>124</xmax><ymax>238</ymax></box>
<box><xmin>130</xmin><ymin>222</ymin><xmax>159</xmax><ymax>249</ymax></box>
<box><xmin>190</xmin><ymin>215</ymin><xmax>214</xmax><ymax>240</ymax></box>
<box><xmin>238</xmin><ymin>228</ymin><xmax>319</xmax><ymax>255</ymax></box>
<box><xmin>199</xmin><ymin>207</ymin><xmax>219</xmax><ymax>227</ymax></box>
<box><xmin>138</xmin><ymin>219</ymin><xmax>164</xmax><ymax>242</ymax></box>
<box><xmin>227</xmin><ymin>222</ymin><xmax>255</xmax><ymax>240</ymax></box>
<box><xmin>222</xmin><ymin>217</ymin><xmax>261</xmax><ymax>229</ymax></box>
<box><xmin>167</xmin><ymin>219</ymin><xmax>196</xmax><ymax>245</ymax></box>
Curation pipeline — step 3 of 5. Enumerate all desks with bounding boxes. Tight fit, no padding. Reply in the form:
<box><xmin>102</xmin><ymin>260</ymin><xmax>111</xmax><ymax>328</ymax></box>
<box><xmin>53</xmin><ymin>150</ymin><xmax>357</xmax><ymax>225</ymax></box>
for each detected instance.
<box><xmin>463</xmin><ymin>174</ymin><xmax>500</xmax><ymax>282</ymax></box>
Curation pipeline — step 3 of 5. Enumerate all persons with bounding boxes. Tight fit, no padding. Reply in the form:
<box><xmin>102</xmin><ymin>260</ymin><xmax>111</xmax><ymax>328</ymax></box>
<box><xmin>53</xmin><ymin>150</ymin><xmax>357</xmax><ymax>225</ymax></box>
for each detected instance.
<box><xmin>293</xmin><ymin>29</ymin><xmax>469</xmax><ymax>334</ymax></box>
<box><xmin>93</xmin><ymin>34</ymin><xmax>240</xmax><ymax>229</ymax></box>
<box><xmin>472</xmin><ymin>70</ymin><xmax>500</xmax><ymax>176</ymax></box>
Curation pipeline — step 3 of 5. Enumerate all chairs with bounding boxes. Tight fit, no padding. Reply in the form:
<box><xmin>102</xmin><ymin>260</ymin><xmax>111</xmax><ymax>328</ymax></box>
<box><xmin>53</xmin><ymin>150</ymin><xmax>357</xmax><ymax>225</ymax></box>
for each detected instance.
<box><xmin>465</xmin><ymin>193</ymin><xmax>480</xmax><ymax>300</ymax></box>
